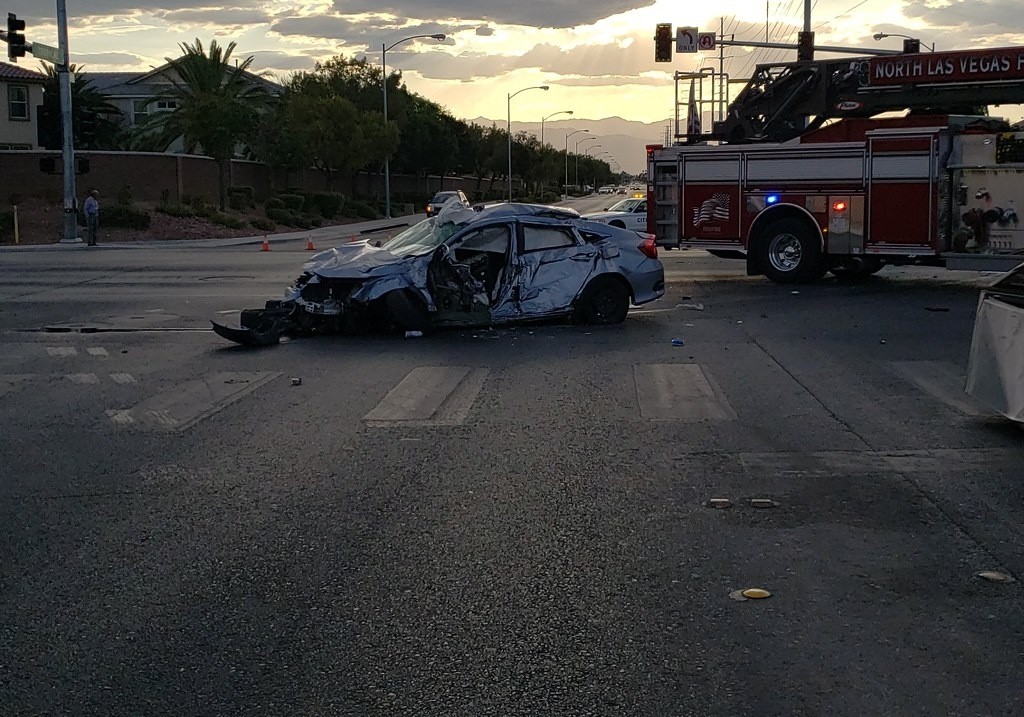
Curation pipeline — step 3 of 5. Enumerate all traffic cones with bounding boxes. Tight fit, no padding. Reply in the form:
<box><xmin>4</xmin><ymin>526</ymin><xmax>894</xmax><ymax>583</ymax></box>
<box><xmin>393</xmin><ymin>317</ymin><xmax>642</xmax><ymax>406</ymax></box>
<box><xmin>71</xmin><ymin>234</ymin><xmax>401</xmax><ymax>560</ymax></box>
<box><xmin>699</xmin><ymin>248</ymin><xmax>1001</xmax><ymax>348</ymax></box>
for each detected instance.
<box><xmin>351</xmin><ymin>234</ymin><xmax>356</xmax><ymax>241</ymax></box>
<box><xmin>305</xmin><ymin>234</ymin><xmax>317</xmax><ymax>251</ymax></box>
<box><xmin>386</xmin><ymin>232</ymin><xmax>391</xmax><ymax>241</ymax></box>
<box><xmin>259</xmin><ymin>234</ymin><xmax>272</xmax><ymax>252</ymax></box>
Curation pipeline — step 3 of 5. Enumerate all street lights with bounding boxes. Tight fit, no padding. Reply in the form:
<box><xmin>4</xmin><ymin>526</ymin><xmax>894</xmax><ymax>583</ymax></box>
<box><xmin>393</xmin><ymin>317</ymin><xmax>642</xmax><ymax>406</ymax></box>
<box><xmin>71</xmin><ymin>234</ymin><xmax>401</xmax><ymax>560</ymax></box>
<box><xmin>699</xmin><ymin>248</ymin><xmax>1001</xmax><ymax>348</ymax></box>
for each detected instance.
<box><xmin>541</xmin><ymin>111</ymin><xmax>574</xmax><ymax>198</ymax></box>
<box><xmin>566</xmin><ymin>130</ymin><xmax>589</xmax><ymax>197</ymax></box>
<box><xmin>586</xmin><ymin>144</ymin><xmax>612</xmax><ymax>163</ymax></box>
<box><xmin>508</xmin><ymin>86</ymin><xmax>549</xmax><ymax>200</ymax></box>
<box><xmin>382</xmin><ymin>34</ymin><xmax>446</xmax><ymax>218</ymax></box>
<box><xmin>576</xmin><ymin>137</ymin><xmax>596</xmax><ymax>187</ymax></box>
<box><xmin>873</xmin><ymin>33</ymin><xmax>936</xmax><ymax>52</ymax></box>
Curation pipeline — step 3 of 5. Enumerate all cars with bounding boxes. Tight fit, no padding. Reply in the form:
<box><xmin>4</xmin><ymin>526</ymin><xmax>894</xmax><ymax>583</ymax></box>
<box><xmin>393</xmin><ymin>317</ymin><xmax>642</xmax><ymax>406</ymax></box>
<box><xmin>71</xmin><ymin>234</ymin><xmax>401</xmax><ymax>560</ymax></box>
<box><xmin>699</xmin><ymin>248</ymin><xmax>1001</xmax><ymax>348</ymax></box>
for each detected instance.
<box><xmin>580</xmin><ymin>197</ymin><xmax>647</xmax><ymax>232</ymax></box>
<box><xmin>597</xmin><ymin>182</ymin><xmax>642</xmax><ymax>196</ymax></box>
<box><xmin>284</xmin><ymin>197</ymin><xmax>665</xmax><ymax>339</ymax></box>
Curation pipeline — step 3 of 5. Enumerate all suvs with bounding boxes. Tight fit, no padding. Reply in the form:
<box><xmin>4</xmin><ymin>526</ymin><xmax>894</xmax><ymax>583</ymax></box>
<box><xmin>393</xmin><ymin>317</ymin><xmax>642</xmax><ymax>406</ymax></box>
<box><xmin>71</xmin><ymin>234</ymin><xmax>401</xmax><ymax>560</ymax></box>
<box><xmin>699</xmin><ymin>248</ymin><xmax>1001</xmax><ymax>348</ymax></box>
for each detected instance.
<box><xmin>428</xmin><ymin>189</ymin><xmax>470</xmax><ymax>219</ymax></box>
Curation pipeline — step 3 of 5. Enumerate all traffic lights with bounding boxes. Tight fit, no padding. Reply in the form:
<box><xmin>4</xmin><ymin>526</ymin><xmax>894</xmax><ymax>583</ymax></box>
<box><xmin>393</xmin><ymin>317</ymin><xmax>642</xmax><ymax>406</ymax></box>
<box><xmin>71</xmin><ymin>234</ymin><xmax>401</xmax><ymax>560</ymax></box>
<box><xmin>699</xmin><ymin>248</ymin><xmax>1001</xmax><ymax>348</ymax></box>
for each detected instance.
<box><xmin>6</xmin><ymin>12</ymin><xmax>26</xmax><ymax>63</ymax></box>
<box><xmin>655</xmin><ymin>24</ymin><xmax>671</xmax><ymax>62</ymax></box>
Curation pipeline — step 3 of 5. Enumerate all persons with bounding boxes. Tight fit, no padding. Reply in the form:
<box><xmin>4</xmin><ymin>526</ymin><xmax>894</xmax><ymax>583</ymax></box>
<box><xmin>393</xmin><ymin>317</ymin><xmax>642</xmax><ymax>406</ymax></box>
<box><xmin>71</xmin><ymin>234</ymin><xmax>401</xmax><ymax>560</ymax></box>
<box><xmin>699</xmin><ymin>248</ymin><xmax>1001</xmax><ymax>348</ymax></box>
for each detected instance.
<box><xmin>84</xmin><ymin>190</ymin><xmax>99</xmax><ymax>246</ymax></box>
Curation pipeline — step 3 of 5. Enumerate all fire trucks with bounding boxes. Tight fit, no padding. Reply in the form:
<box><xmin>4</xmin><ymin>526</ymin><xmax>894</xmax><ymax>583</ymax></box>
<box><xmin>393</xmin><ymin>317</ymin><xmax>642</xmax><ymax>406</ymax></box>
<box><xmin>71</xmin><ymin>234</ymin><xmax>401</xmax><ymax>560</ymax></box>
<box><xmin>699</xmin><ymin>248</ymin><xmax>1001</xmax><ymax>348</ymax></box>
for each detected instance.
<box><xmin>645</xmin><ymin>48</ymin><xmax>1023</xmax><ymax>290</ymax></box>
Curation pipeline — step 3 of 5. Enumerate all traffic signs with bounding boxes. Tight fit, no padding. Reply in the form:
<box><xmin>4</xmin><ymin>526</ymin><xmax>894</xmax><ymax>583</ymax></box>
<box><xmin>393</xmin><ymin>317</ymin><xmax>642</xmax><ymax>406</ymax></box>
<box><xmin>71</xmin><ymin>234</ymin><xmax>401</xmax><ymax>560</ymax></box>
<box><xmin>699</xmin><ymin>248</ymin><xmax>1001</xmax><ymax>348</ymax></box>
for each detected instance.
<box><xmin>677</xmin><ymin>27</ymin><xmax>697</xmax><ymax>53</ymax></box>
